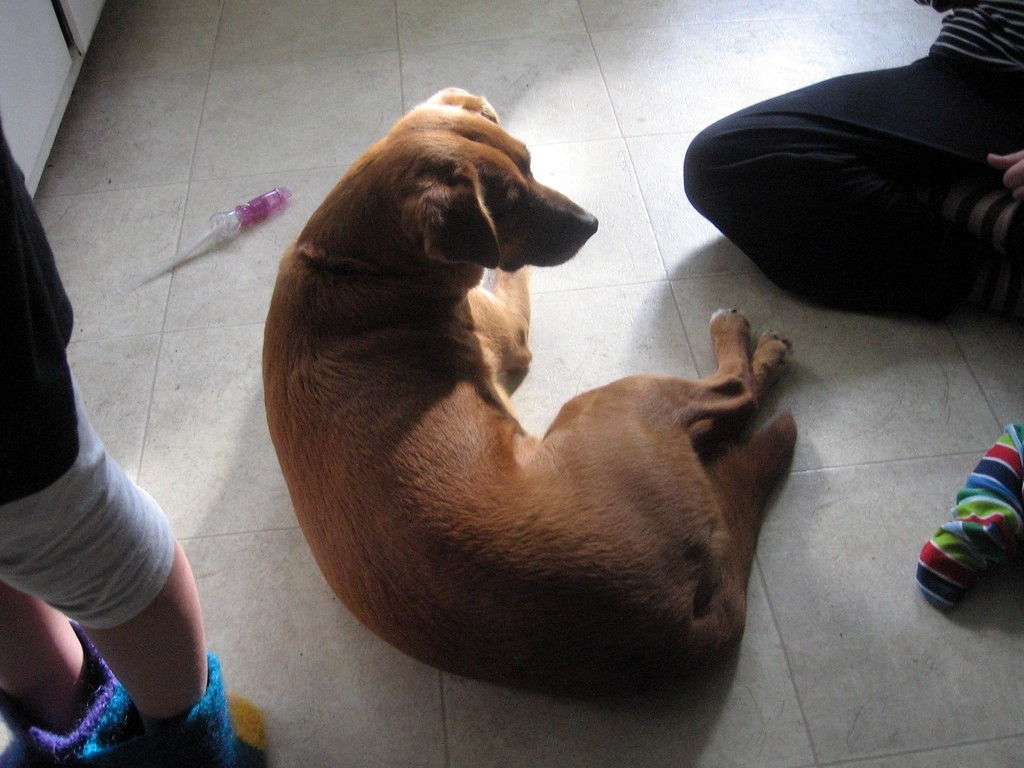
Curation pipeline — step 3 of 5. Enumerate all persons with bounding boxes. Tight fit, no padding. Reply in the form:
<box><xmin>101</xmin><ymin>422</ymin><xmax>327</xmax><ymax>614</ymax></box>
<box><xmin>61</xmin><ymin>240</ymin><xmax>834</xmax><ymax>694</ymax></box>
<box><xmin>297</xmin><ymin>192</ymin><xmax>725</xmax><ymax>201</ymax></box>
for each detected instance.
<box><xmin>0</xmin><ymin>116</ymin><xmax>263</xmax><ymax>768</ymax></box>
<box><xmin>681</xmin><ymin>0</ymin><xmax>1024</xmax><ymax>333</ymax></box>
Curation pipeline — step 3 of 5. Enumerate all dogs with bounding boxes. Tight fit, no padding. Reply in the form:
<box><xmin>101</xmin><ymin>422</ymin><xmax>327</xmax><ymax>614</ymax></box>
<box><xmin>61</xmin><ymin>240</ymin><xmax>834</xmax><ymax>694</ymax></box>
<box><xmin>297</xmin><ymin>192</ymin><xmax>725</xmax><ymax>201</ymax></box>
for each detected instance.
<box><xmin>261</xmin><ymin>86</ymin><xmax>797</xmax><ymax>710</ymax></box>
